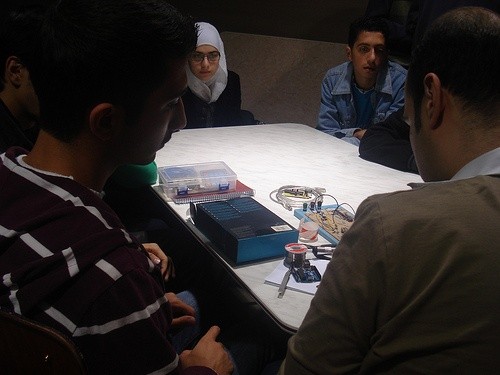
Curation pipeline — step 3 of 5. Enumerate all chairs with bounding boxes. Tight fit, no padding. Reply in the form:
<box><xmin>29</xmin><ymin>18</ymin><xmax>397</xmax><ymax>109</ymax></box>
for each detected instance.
<box><xmin>0</xmin><ymin>310</ymin><xmax>92</xmax><ymax>375</ymax></box>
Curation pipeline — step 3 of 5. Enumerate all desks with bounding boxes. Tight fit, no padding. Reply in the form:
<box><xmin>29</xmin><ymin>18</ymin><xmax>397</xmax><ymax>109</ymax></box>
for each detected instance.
<box><xmin>148</xmin><ymin>123</ymin><xmax>424</xmax><ymax>335</ymax></box>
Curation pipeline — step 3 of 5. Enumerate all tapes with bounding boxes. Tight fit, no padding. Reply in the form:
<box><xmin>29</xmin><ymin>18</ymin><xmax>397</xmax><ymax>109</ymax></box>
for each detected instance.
<box><xmin>284</xmin><ymin>243</ymin><xmax>309</xmax><ymax>268</ymax></box>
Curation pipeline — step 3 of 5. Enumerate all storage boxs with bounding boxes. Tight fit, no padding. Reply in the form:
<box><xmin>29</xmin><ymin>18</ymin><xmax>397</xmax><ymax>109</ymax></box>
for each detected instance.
<box><xmin>157</xmin><ymin>161</ymin><xmax>237</xmax><ymax>197</ymax></box>
<box><xmin>190</xmin><ymin>197</ymin><xmax>299</xmax><ymax>266</ymax></box>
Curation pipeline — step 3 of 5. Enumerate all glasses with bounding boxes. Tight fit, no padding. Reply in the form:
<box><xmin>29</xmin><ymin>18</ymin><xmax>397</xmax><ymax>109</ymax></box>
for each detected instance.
<box><xmin>191</xmin><ymin>51</ymin><xmax>220</xmax><ymax>61</ymax></box>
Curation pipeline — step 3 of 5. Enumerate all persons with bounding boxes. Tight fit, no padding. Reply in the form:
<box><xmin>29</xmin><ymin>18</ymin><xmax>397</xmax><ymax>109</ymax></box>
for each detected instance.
<box><xmin>317</xmin><ymin>17</ymin><xmax>408</xmax><ymax>146</ymax></box>
<box><xmin>359</xmin><ymin>106</ymin><xmax>418</xmax><ymax>175</ymax></box>
<box><xmin>262</xmin><ymin>6</ymin><xmax>500</xmax><ymax>375</ymax></box>
<box><xmin>0</xmin><ymin>0</ymin><xmax>257</xmax><ymax>375</ymax></box>
